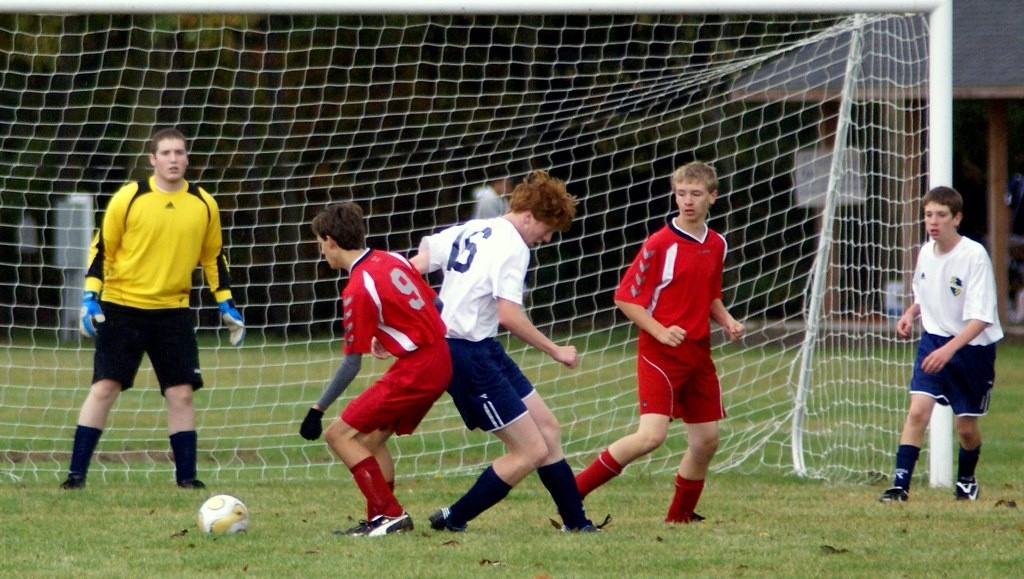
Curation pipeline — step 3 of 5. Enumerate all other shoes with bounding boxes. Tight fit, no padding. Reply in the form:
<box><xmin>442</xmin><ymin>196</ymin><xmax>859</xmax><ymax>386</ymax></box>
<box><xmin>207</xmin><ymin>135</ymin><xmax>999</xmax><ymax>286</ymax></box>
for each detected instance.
<box><xmin>59</xmin><ymin>474</ymin><xmax>83</xmax><ymax>491</ymax></box>
<box><xmin>179</xmin><ymin>480</ymin><xmax>206</xmax><ymax>489</ymax></box>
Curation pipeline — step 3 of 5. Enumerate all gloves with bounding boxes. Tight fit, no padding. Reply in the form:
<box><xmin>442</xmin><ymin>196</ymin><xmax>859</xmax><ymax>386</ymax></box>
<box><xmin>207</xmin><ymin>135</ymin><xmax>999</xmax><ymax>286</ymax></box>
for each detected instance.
<box><xmin>218</xmin><ymin>297</ymin><xmax>246</xmax><ymax>346</ymax></box>
<box><xmin>299</xmin><ymin>407</ymin><xmax>324</xmax><ymax>441</ymax></box>
<box><xmin>79</xmin><ymin>290</ymin><xmax>106</xmax><ymax>337</ymax></box>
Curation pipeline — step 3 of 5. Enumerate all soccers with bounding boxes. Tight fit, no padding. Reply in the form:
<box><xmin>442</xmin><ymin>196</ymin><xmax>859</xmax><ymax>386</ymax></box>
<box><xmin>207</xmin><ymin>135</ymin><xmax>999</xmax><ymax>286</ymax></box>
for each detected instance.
<box><xmin>196</xmin><ymin>494</ymin><xmax>248</xmax><ymax>535</ymax></box>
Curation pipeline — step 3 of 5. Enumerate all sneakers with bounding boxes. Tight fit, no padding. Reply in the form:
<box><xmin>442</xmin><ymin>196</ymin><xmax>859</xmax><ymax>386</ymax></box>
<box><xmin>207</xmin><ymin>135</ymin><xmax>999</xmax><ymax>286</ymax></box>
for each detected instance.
<box><xmin>334</xmin><ymin>511</ymin><xmax>414</xmax><ymax>537</ymax></box>
<box><xmin>560</xmin><ymin>514</ymin><xmax>613</xmax><ymax>531</ymax></box>
<box><xmin>429</xmin><ymin>507</ymin><xmax>467</xmax><ymax>533</ymax></box>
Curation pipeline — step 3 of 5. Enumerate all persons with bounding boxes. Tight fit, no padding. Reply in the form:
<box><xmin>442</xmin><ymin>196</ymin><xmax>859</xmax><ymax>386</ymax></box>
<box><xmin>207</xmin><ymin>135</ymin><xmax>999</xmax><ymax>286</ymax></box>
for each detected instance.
<box><xmin>410</xmin><ymin>172</ymin><xmax>601</xmax><ymax>532</ymax></box>
<box><xmin>58</xmin><ymin>129</ymin><xmax>245</xmax><ymax>491</ymax></box>
<box><xmin>300</xmin><ymin>203</ymin><xmax>453</xmax><ymax>539</ymax></box>
<box><xmin>575</xmin><ymin>161</ymin><xmax>747</xmax><ymax>525</ymax></box>
<box><xmin>876</xmin><ymin>185</ymin><xmax>1004</xmax><ymax>506</ymax></box>
